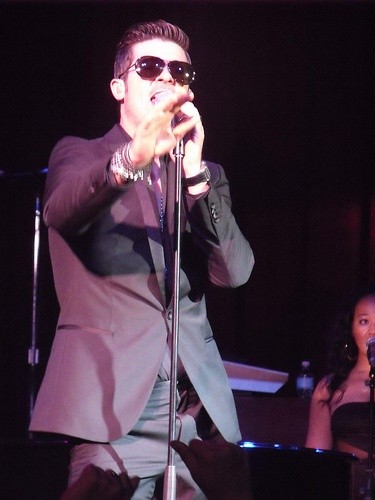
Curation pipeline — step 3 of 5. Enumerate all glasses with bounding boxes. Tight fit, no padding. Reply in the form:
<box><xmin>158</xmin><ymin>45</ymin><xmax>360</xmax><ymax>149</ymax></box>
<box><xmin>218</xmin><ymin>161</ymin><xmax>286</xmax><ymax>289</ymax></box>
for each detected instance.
<box><xmin>118</xmin><ymin>55</ymin><xmax>196</xmax><ymax>86</ymax></box>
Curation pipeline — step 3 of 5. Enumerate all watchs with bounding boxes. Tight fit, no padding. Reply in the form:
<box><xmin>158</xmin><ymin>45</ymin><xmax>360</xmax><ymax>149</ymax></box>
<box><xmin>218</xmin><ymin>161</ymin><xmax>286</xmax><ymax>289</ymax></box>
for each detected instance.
<box><xmin>183</xmin><ymin>159</ymin><xmax>212</xmax><ymax>188</ymax></box>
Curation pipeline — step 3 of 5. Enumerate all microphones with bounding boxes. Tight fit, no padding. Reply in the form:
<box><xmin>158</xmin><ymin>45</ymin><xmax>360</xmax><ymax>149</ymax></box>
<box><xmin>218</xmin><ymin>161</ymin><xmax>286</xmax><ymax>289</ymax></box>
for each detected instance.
<box><xmin>156</xmin><ymin>92</ymin><xmax>189</xmax><ymax>124</ymax></box>
<box><xmin>367</xmin><ymin>338</ymin><xmax>375</xmax><ymax>378</ymax></box>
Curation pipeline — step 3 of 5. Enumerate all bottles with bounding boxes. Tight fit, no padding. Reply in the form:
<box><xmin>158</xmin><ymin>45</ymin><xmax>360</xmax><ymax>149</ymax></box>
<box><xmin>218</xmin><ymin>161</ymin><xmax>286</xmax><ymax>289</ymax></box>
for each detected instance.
<box><xmin>296</xmin><ymin>360</ymin><xmax>314</xmax><ymax>399</ymax></box>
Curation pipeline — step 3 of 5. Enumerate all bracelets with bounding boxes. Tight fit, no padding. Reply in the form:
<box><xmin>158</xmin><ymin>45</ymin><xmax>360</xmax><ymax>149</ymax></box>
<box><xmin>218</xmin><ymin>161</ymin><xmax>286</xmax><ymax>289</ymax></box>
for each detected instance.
<box><xmin>109</xmin><ymin>136</ymin><xmax>155</xmax><ymax>181</ymax></box>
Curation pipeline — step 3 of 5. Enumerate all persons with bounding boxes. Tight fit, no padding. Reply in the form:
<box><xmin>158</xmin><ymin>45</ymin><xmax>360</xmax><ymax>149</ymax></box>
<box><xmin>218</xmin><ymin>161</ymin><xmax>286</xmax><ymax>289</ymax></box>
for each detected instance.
<box><xmin>306</xmin><ymin>286</ymin><xmax>375</xmax><ymax>459</ymax></box>
<box><xmin>27</xmin><ymin>18</ymin><xmax>256</xmax><ymax>500</ymax></box>
